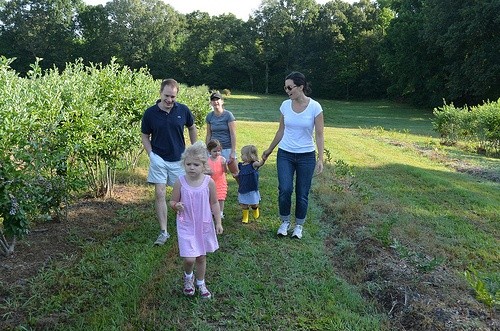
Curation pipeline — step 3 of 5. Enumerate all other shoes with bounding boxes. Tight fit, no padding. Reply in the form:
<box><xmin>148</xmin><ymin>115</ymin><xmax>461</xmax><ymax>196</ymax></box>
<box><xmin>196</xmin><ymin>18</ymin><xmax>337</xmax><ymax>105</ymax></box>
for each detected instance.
<box><xmin>220</xmin><ymin>214</ymin><xmax>225</xmax><ymax>220</ymax></box>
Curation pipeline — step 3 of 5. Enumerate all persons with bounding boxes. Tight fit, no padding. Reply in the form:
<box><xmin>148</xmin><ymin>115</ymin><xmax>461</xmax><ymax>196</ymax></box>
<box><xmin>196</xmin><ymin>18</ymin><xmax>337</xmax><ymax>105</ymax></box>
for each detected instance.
<box><xmin>263</xmin><ymin>71</ymin><xmax>324</xmax><ymax>238</ymax></box>
<box><xmin>170</xmin><ymin>141</ymin><xmax>223</xmax><ymax>297</ymax></box>
<box><xmin>231</xmin><ymin>144</ymin><xmax>265</xmax><ymax>223</ymax></box>
<box><xmin>205</xmin><ymin>93</ymin><xmax>239</xmax><ymax>178</ymax></box>
<box><xmin>140</xmin><ymin>79</ymin><xmax>197</xmax><ymax>246</ymax></box>
<box><xmin>207</xmin><ymin>139</ymin><xmax>227</xmax><ymax>220</ymax></box>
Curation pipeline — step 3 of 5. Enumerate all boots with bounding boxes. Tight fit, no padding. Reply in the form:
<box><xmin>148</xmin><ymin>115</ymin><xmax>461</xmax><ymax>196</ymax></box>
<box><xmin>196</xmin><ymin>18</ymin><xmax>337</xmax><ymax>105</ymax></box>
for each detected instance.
<box><xmin>253</xmin><ymin>208</ymin><xmax>260</xmax><ymax>218</ymax></box>
<box><xmin>242</xmin><ymin>209</ymin><xmax>249</xmax><ymax>224</ymax></box>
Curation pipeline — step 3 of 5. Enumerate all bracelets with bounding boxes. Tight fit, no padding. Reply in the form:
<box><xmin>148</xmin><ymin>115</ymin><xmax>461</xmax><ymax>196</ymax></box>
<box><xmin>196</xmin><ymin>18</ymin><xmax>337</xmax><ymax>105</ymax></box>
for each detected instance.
<box><xmin>174</xmin><ymin>203</ymin><xmax>183</xmax><ymax>223</ymax></box>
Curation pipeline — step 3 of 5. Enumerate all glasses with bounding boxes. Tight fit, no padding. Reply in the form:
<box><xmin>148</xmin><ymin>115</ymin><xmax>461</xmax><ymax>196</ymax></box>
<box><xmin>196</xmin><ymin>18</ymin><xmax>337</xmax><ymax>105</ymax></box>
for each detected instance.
<box><xmin>284</xmin><ymin>85</ymin><xmax>297</xmax><ymax>91</ymax></box>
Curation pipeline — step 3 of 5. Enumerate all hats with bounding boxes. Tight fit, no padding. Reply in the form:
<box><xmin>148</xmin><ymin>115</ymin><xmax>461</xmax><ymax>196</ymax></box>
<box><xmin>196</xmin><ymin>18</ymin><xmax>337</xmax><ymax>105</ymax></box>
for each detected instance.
<box><xmin>210</xmin><ymin>93</ymin><xmax>222</xmax><ymax>99</ymax></box>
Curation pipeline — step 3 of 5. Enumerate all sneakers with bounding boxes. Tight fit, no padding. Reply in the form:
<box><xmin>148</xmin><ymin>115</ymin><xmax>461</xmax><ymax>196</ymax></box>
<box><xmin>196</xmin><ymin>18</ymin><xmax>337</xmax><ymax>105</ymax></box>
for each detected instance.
<box><xmin>184</xmin><ymin>274</ymin><xmax>196</xmax><ymax>296</ymax></box>
<box><xmin>153</xmin><ymin>230</ymin><xmax>171</xmax><ymax>246</ymax></box>
<box><xmin>277</xmin><ymin>221</ymin><xmax>291</xmax><ymax>236</ymax></box>
<box><xmin>291</xmin><ymin>225</ymin><xmax>303</xmax><ymax>239</ymax></box>
<box><xmin>197</xmin><ymin>283</ymin><xmax>212</xmax><ymax>298</ymax></box>
